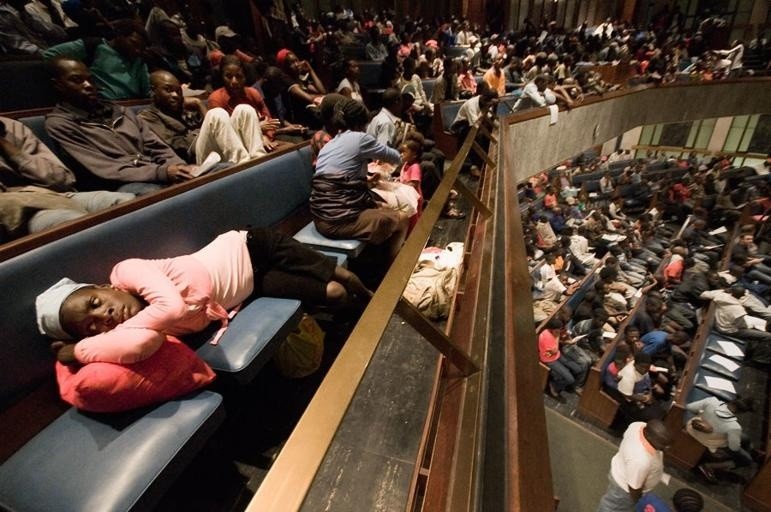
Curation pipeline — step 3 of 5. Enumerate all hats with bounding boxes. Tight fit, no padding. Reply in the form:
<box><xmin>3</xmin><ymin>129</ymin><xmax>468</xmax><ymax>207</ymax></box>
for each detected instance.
<box><xmin>215</xmin><ymin>25</ymin><xmax>236</xmax><ymax>39</ymax></box>
<box><xmin>276</xmin><ymin>47</ymin><xmax>293</xmax><ymax>66</ymax></box>
<box><xmin>33</xmin><ymin>275</ymin><xmax>97</xmax><ymax>341</ymax></box>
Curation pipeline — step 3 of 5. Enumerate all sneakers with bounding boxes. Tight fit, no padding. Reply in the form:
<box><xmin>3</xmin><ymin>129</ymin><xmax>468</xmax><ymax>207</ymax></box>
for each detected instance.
<box><xmin>698</xmin><ymin>463</ymin><xmax>718</xmax><ymax>483</ymax></box>
<box><xmin>753</xmin><ymin>447</ymin><xmax>765</xmax><ymax>458</ymax></box>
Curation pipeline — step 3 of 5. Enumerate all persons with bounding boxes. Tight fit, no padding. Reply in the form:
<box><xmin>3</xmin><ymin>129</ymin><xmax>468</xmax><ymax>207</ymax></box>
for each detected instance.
<box><xmin>0</xmin><ymin>1</ymin><xmax>295</xmax><ymax>245</ymax></box>
<box><xmin>270</xmin><ymin>0</ymin><xmax>771</xmax><ymax>284</ymax></box>
<box><xmin>517</xmin><ymin>147</ymin><xmax>769</xmax><ymax>481</ymax></box>
<box><xmin>634</xmin><ymin>489</ymin><xmax>703</xmax><ymax>512</ymax></box>
<box><xmin>34</xmin><ymin>228</ymin><xmax>377</xmax><ymax>363</ymax></box>
<box><xmin>597</xmin><ymin>419</ymin><xmax>671</xmax><ymax>512</ymax></box>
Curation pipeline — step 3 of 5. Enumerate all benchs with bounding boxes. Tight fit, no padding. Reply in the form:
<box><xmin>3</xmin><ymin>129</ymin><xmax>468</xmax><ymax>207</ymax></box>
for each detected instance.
<box><xmin>2</xmin><ymin>3</ymin><xmax>426</xmax><ymax>509</ymax></box>
<box><xmin>429</xmin><ymin>4</ymin><xmax>761</xmax><ymax>88</ymax></box>
<box><xmin>521</xmin><ymin>150</ymin><xmax>767</xmax><ymax>507</ymax></box>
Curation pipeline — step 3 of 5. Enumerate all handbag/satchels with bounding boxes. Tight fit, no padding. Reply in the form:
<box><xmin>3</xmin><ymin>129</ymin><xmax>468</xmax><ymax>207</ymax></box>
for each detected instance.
<box><xmin>403</xmin><ymin>260</ymin><xmax>457</xmax><ymax>321</ymax></box>
<box><xmin>273</xmin><ymin>312</ymin><xmax>327</xmax><ymax>379</ymax></box>
